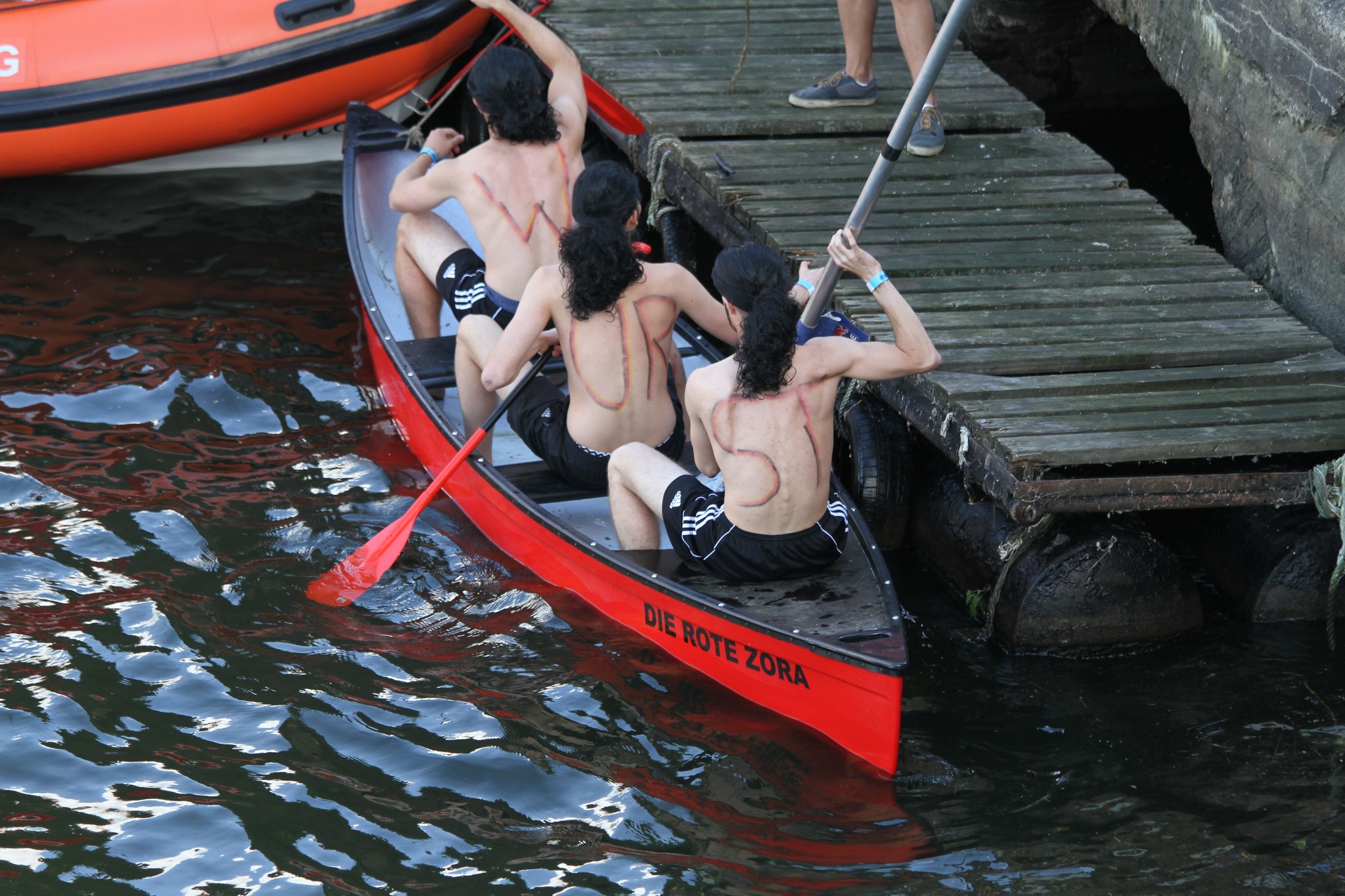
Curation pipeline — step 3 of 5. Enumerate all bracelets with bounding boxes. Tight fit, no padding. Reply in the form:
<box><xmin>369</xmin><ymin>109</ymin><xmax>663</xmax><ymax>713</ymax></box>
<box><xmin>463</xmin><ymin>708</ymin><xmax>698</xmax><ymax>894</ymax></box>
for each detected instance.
<box><xmin>417</xmin><ymin>147</ymin><xmax>437</xmax><ymax>167</ymax></box>
<box><xmin>796</xmin><ymin>280</ymin><xmax>814</xmax><ymax>298</ymax></box>
<box><xmin>866</xmin><ymin>271</ymin><xmax>888</xmax><ymax>293</ymax></box>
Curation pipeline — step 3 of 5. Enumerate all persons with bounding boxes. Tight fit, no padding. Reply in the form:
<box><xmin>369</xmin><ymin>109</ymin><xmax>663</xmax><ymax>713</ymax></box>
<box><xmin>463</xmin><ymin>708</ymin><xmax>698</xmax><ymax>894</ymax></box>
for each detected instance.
<box><xmin>788</xmin><ymin>1</ymin><xmax>947</xmax><ymax>157</ymax></box>
<box><xmin>453</xmin><ymin>160</ymin><xmax>829</xmax><ymax>477</ymax></box>
<box><xmin>606</xmin><ymin>227</ymin><xmax>942</xmax><ymax>585</ymax></box>
<box><xmin>386</xmin><ymin>0</ymin><xmax>589</xmax><ymax>401</ymax></box>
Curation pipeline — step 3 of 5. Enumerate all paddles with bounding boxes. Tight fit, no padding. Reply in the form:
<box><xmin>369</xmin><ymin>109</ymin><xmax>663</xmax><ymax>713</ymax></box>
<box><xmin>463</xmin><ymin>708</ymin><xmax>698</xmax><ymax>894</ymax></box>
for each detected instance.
<box><xmin>793</xmin><ymin>0</ymin><xmax>973</xmax><ymax>347</ymax></box>
<box><xmin>305</xmin><ymin>345</ymin><xmax>555</xmax><ymax>607</ymax></box>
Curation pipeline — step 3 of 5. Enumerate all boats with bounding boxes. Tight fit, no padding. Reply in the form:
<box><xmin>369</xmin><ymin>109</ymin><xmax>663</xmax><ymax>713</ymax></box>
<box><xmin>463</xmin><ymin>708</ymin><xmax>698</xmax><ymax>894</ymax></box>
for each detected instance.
<box><xmin>339</xmin><ymin>99</ymin><xmax>908</xmax><ymax>782</ymax></box>
<box><xmin>0</xmin><ymin>1</ymin><xmax>546</xmax><ymax>183</ymax></box>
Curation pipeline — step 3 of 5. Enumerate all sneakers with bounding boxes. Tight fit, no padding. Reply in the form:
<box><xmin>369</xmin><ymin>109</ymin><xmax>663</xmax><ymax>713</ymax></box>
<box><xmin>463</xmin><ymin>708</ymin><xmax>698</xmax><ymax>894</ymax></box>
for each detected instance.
<box><xmin>789</xmin><ymin>69</ymin><xmax>878</xmax><ymax>108</ymax></box>
<box><xmin>907</xmin><ymin>100</ymin><xmax>946</xmax><ymax>156</ymax></box>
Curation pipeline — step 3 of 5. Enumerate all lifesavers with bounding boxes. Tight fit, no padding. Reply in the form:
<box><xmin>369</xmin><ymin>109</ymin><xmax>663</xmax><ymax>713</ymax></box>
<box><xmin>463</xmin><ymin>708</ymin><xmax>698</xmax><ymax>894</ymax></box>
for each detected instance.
<box><xmin>832</xmin><ymin>389</ymin><xmax>921</xmax><ymax>554</ymax></box>
<box><xmin>636</xmin><ymin>198</ymin><xmax>716</xmax><ymax>304</ymax></box>
